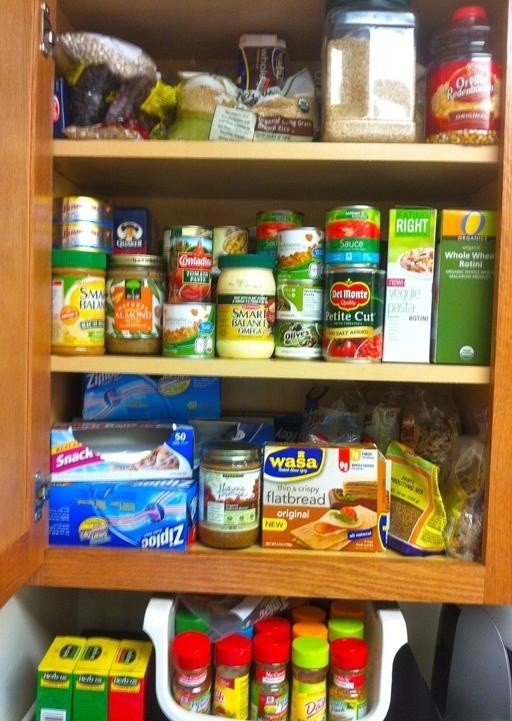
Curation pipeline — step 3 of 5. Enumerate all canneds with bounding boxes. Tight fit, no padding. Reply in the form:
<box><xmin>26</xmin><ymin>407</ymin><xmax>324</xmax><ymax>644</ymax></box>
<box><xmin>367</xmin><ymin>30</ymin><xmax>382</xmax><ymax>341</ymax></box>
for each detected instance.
<box><xmin>254</xmin><ymin>210</ymin><xmax>305</xmax><ymax>258</ymax></box>
<box><xmin>216</xmin><ymin>254</ymin><xmax>276</xmax><ymax>358</ymax></box>
<box><xmin>325</xmin><ymin>205</ymin><xmax>381</xmax><ymax>269</ymax></box>
<box><xmin>212</xmin><ymin>224</ymin><xmax>249</xmax><ymax>275</ymax></box>
<box><xmin>276</xmin><ymin>226</ymin><xmax>326</xmax><ymax>287</ymax></box>
<box><xmin>51</xmin><ymin>249</ymin><xmax>105</xmax><ymax>356</ymax></box>
<box><xmin>322</xmin><ymin>267</ymin><xmax>385</xmax><ymax>364</ymax></box>
<box><xmin>275</xmin><ymin>279</ymin><xmax>323</xmax><ymax>360</ymax></box>
<box><xmin>106</xmin><ymin>253</ymin><xmax>165</xmax><ymax>356</ymax></box>
<box><xmin>53</xmin><ymin>196</ymin><xmax>113</xmax><ymax>252</ymax></box>
<box><xmin>162</xmin><ymin>302</ymin><xmax>216</xmax><ymax>359</ymax></box>
<box><xmin>198</xmin><ymin>445</ymin><xmax>262</xmax><ymax>551</ymax></box>
<box><xmin>162</xmin><ymin>224</ymin><xmax>213</xmax><ymax>302</ymax></box>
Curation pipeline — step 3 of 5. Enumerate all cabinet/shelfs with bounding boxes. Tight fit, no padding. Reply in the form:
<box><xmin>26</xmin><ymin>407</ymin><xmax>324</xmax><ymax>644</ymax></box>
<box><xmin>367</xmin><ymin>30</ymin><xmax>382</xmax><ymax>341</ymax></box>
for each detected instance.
<box><xmin>35</xmin><ymin>2</ymin><xmax>510</xmax><ymax>610</ymax></box>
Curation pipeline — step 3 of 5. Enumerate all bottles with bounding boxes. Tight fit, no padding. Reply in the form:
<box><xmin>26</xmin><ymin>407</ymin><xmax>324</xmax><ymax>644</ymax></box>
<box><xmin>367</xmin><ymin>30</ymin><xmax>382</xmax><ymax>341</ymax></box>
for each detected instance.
<box><xmin>317</xmin><ymin>0</ymin><xmax>418</xmax><ymax>144</ymax></box>
<box><xmin>170</xmin><ymin>594</ymin><xmax>369</xmax><ymax>721</ymax></box>
<box><xmin>423</xmin><ymin>4</ymin><xmax>503</xmax><ymax>146</ymax></box>
